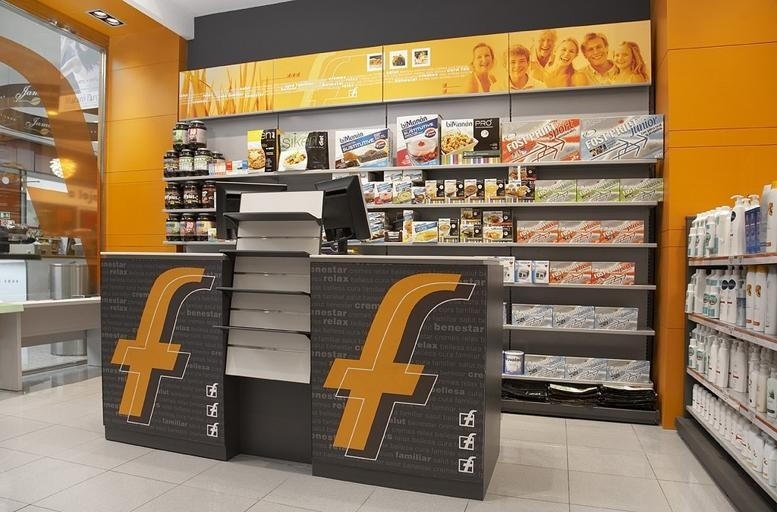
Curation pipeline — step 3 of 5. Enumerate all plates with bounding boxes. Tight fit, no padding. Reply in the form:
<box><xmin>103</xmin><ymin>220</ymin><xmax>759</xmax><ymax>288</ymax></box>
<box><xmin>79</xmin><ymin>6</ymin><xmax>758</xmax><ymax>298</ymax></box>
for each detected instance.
<box><xmin>441</xmin><ymin>134</ymin><xmax>479</xmax><ymax>157</ymax></box>
<box><xmin>483</xmin><ymin>214</ymin><xmax>506</xmax><ymax>241</ymax></box>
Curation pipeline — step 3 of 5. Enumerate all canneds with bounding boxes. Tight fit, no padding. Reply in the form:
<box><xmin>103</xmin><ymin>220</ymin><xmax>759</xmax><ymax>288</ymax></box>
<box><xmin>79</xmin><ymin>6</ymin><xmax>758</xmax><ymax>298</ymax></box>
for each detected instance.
<box><xmin>163</xmin><ymin>120</ymin><xmax>226</xmax><ymax>241</ymax></box>
<box><xmin>504</xmin><ymin>350</ymin><xmax>524</xmax><ymax>375</ymax></box>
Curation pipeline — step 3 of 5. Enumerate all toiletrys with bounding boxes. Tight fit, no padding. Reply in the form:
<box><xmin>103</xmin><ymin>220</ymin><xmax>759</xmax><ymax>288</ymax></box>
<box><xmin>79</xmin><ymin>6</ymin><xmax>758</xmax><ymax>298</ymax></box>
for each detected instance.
<box><xmin>692</xmin><ymin>383</ymin><xmax>777</xmax><ymax>487</ymax></box>
<box><xmin>719</xmin><ymin>265</ymin><xmax>777</xmax><ymax>335</ymax></box>
<box><xmin>759</xmin><ymin>180</ymin><xmax>777</xmax><ymax>253</ymax></box>
<box><xmin>708</xmin><ymin>333</ymin><xmax>776</xmax><ymax>418</ymax></box>
<box><xmin>729</xmin><ymin>194</ymin><xmax>760</xmax><ymax>256</ymax></box>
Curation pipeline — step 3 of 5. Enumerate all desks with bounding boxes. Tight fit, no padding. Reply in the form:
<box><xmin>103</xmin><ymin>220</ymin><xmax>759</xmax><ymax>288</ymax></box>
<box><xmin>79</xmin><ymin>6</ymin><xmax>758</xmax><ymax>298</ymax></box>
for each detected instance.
<box><xmin>0</xmin><ymin>296</ymin><xmax>101</xmax><ymax>392</ymax></box>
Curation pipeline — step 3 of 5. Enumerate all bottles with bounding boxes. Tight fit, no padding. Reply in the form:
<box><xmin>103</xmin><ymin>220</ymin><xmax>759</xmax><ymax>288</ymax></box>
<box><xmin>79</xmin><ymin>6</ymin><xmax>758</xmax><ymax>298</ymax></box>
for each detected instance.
<box><xmin>686</xmin><ymin>322</ymin><xmax>728</xmax><ymax>375</ymax></box>
<box><xmin>687</xmin><ymin>204</ymin><xmax>731</xmax><ymax>256</ymax></box>
<box><xmin>683</xmin><ymin>266</ymin><xmax>724</xmax><ymax>319</ymax></box>
<box><xmin>760</xmin><ymin>180</ymin><xmax>777</xmax><ymax>253</ymax></box>
<box><xmin>744</xmin><ymin>264</ymin><xmax>777</xmax><ymax>336</ymax></box>
<box><xmin>690</xmin><ymin>382</ymin><xmax>777</xmax><ymax>489</ymax></box>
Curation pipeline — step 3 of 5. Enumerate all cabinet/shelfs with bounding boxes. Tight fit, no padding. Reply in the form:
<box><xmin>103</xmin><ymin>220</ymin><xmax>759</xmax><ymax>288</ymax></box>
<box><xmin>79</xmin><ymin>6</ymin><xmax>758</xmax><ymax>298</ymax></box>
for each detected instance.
<box><xmin>230</xmin><ymin>82</ymin><xmax>658</xmax><ymax>247</ymax></box>
<box><xmin>165</xmin><ymin>118</ymin><xmax>230</xmax><ymax>243</ymax></box>
<box><xmin>101</xmin><ymin>252</ymin><xmax>500</xmax><ymax>500</ymax></box>
<box><xmin>498</xmin><ymin>256</ymin><xmax>660</xmax><ymax>425</ymax></box>
<box><xmin>674</xmin><ymin>216</ymin><xmax>776</xmax><ymax>507</ymax></box>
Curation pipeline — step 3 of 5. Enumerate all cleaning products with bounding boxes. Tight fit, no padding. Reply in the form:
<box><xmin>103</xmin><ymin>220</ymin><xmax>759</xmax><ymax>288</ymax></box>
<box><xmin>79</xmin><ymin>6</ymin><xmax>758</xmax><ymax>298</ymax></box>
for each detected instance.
<box><xmin>687</xmin><ymin>205</ymin><xmax>732</xmax><ymax>257</ymax></box>
<box><xmin>687</xmin><ymin>323</ymin><xmax>718</xmax><ymax>376</ymax></box>
<box><xmin>683</xmin><ymin>268</ymin><xmax>725</xmax><ymax>319</ymax></box>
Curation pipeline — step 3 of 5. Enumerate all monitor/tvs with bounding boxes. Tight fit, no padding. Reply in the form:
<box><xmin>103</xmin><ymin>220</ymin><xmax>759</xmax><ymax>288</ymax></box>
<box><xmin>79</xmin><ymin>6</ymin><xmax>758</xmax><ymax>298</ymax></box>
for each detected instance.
<box><xmin>214</xmin><ymin>180</ymin><xmax>288</xmax><ymax>240</ymax></box>
<box><xmin>314</xmin><ymin>174</ymin><xmax>372</xmax><ymax>254</ymax></box>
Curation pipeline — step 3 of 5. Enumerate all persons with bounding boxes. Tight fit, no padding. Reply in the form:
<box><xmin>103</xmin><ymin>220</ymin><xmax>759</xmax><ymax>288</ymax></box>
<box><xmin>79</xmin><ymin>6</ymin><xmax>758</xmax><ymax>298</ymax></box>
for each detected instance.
<box><xmin>459</xmin><ymin>29</ymin><xmax>649</xmax><ymax>93</ymax></box>
